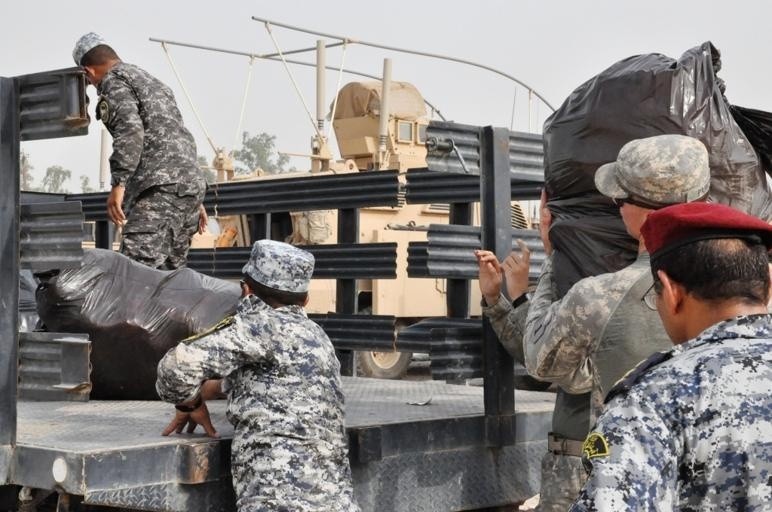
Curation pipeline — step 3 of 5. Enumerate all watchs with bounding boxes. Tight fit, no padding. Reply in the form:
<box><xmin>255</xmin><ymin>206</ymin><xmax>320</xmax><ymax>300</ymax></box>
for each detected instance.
<box><xmin>513</xmin><ymin>291</ymin><xmax>533</xmax><ymax>309</ymax></box>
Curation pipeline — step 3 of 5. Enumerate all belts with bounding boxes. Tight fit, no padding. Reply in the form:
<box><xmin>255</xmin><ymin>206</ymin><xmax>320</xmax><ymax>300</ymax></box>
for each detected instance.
<box><xmin>547</xmin><ymin>430</ymin><xmax>583</xmax><ymax>458</ymax></box>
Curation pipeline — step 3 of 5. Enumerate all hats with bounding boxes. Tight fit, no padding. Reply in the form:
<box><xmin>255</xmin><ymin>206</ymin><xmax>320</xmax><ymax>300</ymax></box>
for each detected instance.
<box><xmin>641</xmin><ymin>202</ymin><xmax>771</xmax><ymax>258</ymax></box>
<box><xmin>244</xmin><ymin>239</ymin><xmax>315</xmax><ymax>294</ymax></box>
<box><xmin>594</xmin><ymin>135</ymin><xmax>712</xmax><ymax>204</ymax></box>
<box><xmin>73</xmin><ymin>31</ymin><xmax>108</xmax><ymax>64</ymax></box>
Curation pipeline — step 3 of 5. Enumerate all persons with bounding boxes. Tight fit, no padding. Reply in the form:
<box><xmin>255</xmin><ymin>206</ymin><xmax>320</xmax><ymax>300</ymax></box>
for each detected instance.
<box><xmin>521</xmin><ymin>134</ymin><xmax>711</xmax><ymax>436</ymax></box>
<box><xmin>471</xmin><ymin>238</ymin><xmax>591</xmax><ymax>512</ymax></box>
<box><xmin>566</xmin><ymin>201</ymin><xmax>772</xmax><ymax>512</ymax></box>
<box><xmin>72</xmin><ymin>32</ymin><xmax>209</xmax><ymax>273</ymax></box>
<box><xmin>154</xmin><ymin>239</ymin><xmax>364</xmax><ymax>512</ymax></box>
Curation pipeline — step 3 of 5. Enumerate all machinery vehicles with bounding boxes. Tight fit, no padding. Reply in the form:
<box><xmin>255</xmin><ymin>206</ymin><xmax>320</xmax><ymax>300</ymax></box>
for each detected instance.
<box><xmin>149</xmin><ymin>13</ymin><xmax>556</xmax><ymax>390</ymax></box>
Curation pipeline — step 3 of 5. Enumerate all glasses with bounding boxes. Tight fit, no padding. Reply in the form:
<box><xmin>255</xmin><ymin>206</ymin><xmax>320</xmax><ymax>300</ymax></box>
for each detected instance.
<box><xmin>640</xmin><ymin>269</ymin><xmax>671</xmax><ymax>311</ymax></box>
<box><xmin>614</xmin><ymin>197</ymin><xmax>655</xmax><ymax>210</ymax></box>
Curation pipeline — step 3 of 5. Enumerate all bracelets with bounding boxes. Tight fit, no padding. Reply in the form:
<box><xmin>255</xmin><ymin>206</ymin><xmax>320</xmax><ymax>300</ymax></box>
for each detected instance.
<box><xmin>173</xmin><ymin>396</ymin><xmax>203</xmax><ymax>413</ymax></box>
